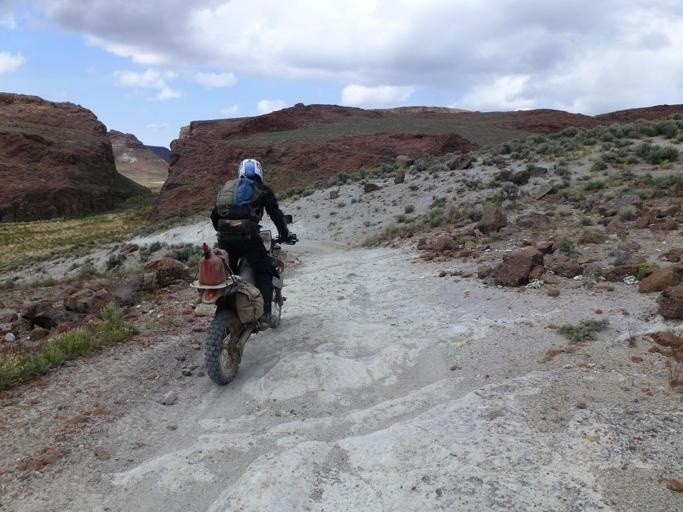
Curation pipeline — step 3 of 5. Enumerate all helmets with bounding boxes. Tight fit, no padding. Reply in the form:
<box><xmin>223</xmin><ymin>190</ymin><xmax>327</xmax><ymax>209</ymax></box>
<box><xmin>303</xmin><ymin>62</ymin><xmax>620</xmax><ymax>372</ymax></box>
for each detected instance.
<box><xmin>239</xmin><ymin>158</ymin><xmax>264</xmax><ymax>183</ymax></box>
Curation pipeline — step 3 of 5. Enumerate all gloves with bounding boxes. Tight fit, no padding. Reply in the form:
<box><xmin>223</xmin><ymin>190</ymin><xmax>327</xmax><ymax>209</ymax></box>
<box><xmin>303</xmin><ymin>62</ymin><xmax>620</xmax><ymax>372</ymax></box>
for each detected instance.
<box><xmin>284</xmin><ymin>232</ymin><xmax>296</xmax><ymax>245</ymax></box>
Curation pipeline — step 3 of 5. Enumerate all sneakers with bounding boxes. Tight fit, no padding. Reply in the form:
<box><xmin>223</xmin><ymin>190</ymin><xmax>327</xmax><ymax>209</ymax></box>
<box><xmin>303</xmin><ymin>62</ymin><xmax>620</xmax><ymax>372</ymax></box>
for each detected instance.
<box><xmin>260</xmin><ymin>315</ymin><xmax>277</xmax><ymax>330</ymax></box>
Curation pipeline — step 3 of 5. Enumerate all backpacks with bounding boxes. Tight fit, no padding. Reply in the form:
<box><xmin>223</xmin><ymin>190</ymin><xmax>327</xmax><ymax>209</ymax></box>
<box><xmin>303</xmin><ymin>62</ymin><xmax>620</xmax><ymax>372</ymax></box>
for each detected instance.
<box><xmin>216</xmin><ymin>176</ymin><xmax>260</xmax><ymax>221</ymax></box>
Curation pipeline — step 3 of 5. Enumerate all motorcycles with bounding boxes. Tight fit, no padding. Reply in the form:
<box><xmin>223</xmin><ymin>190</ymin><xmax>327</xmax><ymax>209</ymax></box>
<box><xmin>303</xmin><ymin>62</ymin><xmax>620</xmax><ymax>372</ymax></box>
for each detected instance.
<box><xmin>188</xmin><ymin>215</ymin><xmax>300</xmax><ymax>386</ymax></box>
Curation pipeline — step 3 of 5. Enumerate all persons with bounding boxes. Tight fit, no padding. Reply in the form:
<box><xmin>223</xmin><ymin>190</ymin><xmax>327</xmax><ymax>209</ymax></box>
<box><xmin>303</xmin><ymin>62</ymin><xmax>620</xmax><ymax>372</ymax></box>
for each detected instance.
<box><xmin>208</xmin><ymin>158</ymin><xmax>298</xmax><ymax>317</ymax></box>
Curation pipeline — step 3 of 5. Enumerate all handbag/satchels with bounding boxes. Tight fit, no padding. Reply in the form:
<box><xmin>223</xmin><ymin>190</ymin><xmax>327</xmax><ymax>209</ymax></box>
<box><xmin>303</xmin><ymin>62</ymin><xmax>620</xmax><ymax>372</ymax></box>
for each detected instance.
<box><xmin>215</xmin><ymin>219</ymin><xmax>253</xmax><ymax>242</ymax></box>
<box><xmin>234</xmin><ymin>280</ymin><xmax>265</xmax><ymax>323</ymax></box>
